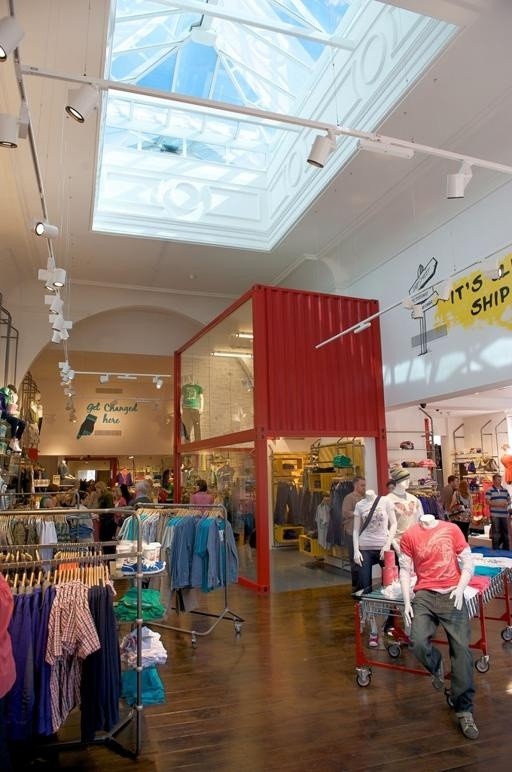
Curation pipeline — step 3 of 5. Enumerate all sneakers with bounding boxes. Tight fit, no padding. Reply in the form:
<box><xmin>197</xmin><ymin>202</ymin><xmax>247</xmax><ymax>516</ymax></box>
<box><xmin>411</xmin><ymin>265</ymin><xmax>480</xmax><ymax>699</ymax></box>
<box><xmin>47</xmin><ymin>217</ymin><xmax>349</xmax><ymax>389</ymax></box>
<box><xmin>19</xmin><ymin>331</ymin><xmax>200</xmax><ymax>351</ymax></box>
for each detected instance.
<box><xmin>456</xmin><ymin>712</ymin><xmax>479</xmax><ymax>739</ymax></box>
<box><xmin>432</xmin><ymin>660</ymin><xmax>444</xmax><ymax>688</ymax></box>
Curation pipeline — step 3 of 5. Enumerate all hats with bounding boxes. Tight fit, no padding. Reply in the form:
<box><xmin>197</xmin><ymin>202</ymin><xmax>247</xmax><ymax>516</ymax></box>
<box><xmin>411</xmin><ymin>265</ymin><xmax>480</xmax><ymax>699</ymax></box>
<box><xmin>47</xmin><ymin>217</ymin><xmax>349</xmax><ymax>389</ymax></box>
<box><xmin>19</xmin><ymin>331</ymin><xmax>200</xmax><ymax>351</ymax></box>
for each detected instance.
<box><xmin>391</xmin><ymin>469</ymin><xmax>410</xmax><ymax>482</ymax></box>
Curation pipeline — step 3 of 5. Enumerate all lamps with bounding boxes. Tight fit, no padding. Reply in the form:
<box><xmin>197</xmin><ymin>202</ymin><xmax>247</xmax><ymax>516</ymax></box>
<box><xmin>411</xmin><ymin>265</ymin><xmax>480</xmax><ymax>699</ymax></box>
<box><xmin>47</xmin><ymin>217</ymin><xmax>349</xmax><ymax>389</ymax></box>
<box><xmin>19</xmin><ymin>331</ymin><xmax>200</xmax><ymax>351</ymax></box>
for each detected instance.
<box><xmin>35</xmin><ymin>216</ymin><xmax>75</xmax><ymax>400</ymax></box>
<box><xmin>98</xmin><ymin>374</ymin><xmax>164</xmax><ymax>390</ymax></box>
<box><xmin>0</xmin><ymin>16</ymin><xmax>108</xmax><ymax>160</ymax></box>
<box><xmin>400</xmin><ymin>257</ymin><xmax>506</xmax><ymax>320</ymax></box>
<box><xmin>209</xmin><ymin>332</ymin><xmax>253</xmax><ymax>359</ymax></box>
<box><xmin>306</xmin><ymin>117</ymin><xmax>479</xmax><ymax>209</ymax></box>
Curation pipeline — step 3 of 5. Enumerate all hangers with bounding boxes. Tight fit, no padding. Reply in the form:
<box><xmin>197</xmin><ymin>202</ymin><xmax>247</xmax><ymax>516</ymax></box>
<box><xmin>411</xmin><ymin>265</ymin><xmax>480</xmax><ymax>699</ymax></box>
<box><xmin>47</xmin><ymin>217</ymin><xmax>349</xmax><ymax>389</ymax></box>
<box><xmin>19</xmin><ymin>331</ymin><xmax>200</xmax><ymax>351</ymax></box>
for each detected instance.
<box><xmin>1</xmin><ymin>506</ymin><xmax>118</xmax><ymax>598</ymax></box>
<box><xmin>137</xmin><ymin>507</ymin><xmax>222</xmax><ymax>527</ymax></box>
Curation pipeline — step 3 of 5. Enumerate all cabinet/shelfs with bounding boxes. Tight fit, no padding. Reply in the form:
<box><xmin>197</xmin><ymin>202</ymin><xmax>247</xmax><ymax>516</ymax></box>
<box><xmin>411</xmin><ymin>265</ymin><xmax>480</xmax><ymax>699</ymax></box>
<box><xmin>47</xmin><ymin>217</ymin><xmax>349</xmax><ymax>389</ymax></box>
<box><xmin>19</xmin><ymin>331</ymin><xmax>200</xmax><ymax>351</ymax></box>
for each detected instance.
<box><xmin>388</xmin><ymin>447</ymin><xmax>498</xmax><ymax>491</ymax></box>
<box><xmin>270</xmin><ymin>439</ymin><xmax>364</xmax><ymax>558</ymax></box>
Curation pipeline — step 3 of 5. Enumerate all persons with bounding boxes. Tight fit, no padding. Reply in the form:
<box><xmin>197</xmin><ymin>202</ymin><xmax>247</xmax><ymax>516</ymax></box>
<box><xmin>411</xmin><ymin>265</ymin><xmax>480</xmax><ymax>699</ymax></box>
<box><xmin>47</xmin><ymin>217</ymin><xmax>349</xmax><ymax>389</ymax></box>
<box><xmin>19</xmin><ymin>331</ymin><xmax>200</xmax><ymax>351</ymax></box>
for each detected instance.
<box><xmin>340</xmin><ymin>476</ymin><xmax>367</xmax><ymax>593</ymax></box>
<box><xmin>478</xmin><ymin>452</ymin><xmax>499</xmax><ymax>472</ymax></box>
<box><xmin>189</xmin><ymin>479</ymin><xmax>214</xmax><ymax>511</ymax></box>
<box><xmin>501</xmin><ymin>444</ymin><xmax>512</xmax><ymax>485</ymax></box>
<box><xmin>384</xmin><ymin>459</ymin><xmax>423</xmax><ymax>570</ymax></box>
<box><xmin>180</xmin><ymin>379</ymin><xmax>204</xmax><ymax>443</ymax></box>
<box><xmin>30</xmin><ymin>391</ymin><xmax>43</xmax><ymax>436</ymax></box>
<box><xmin>440</xmin><ymin>474</ymin><xmax>459</xmax><ymax>511</ymax></box>
<box><xmin>350</xmin><ymin>488</ymin><xmax>397</xmax><ymax>648</ymax></box>
<box><xmin>398</xmin><ymin>512</ymin><xmax>480</xmax><ymax>742</ymax></box>
<box><xmin>0</xmin><ymin>385</ymin><xmax>26</xmax><ymax>453</ymax></box>
<box><xmin>35</xmin><ymin>473</ymin><xmax>174</xmax><ymax>569</ymax></box>
<box><xmin>484</xmin><ymin>473</ymin><xmax>512</xmax><ymax>550</ymax></box>
<box><xmin>446</xmin><ymin>479</ymin><xmax>472</xmax><ymax>544</ymax></box>
<box><xmin>59</xmin><ymin>459</ymin><xmax>69</xmax><ymax>478</ymax></box>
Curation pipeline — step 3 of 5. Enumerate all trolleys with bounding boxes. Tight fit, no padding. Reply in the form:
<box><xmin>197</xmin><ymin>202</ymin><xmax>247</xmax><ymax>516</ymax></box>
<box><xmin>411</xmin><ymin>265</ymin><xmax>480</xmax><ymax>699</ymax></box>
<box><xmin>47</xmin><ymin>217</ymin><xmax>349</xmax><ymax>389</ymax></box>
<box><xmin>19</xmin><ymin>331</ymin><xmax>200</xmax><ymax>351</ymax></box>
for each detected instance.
<box><xmin>350</xmin><ymin>549</ymin><xmax>512</xmax><ymax>709</ymax></box>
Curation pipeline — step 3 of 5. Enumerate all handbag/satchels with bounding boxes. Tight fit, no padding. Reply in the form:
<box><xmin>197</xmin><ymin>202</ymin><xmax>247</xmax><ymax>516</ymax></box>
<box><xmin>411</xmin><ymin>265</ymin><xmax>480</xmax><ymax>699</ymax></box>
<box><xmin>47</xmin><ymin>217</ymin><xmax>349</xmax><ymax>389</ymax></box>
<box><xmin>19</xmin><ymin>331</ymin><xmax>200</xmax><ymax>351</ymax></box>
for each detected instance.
<box><xmin>452</xmin><ymin>504</ymin><xmax>465</xmax><ymax>514</ymax></box>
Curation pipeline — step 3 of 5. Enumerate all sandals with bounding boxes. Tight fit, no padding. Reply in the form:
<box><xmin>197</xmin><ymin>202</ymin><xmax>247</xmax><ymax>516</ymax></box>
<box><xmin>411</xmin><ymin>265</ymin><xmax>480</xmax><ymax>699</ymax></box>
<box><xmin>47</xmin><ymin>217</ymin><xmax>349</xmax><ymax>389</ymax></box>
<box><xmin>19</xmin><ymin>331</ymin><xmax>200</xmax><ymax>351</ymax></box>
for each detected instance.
<box><xmin>370</xmin><ymin>633</ymin><xmax>378</xmax><ymax>647</ymax></box>
